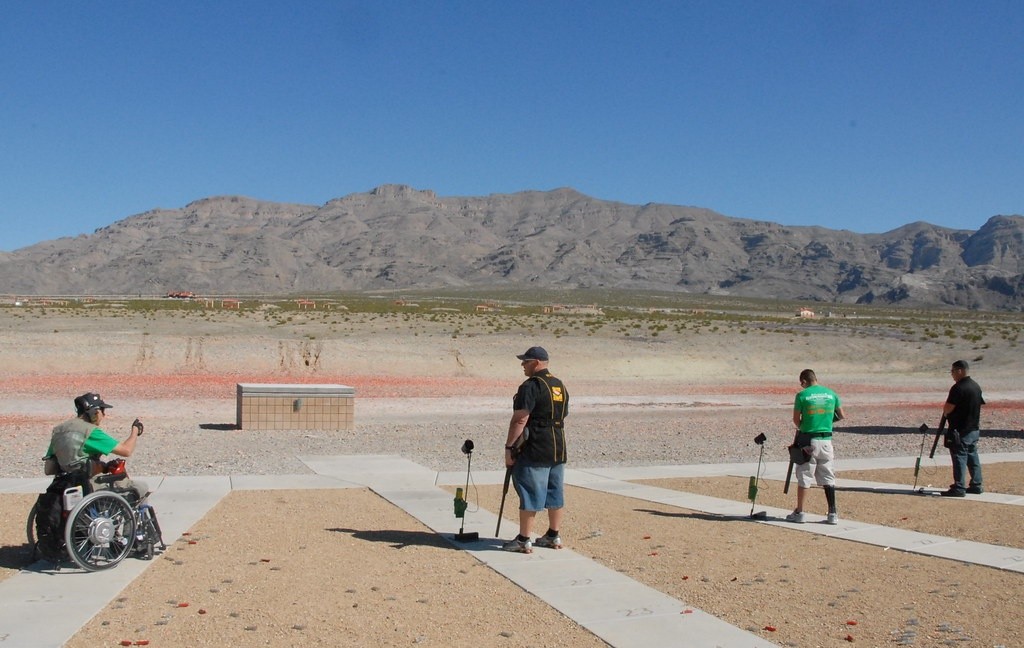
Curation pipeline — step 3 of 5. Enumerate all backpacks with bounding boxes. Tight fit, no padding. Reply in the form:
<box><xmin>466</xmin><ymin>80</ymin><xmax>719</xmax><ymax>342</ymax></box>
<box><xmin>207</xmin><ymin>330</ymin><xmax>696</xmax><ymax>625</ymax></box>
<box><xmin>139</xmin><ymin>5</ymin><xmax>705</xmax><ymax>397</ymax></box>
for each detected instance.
<box><xmin>37</xmin><ymin>470</ymin><xmax>96</xmax><ymax>558</ymax></box>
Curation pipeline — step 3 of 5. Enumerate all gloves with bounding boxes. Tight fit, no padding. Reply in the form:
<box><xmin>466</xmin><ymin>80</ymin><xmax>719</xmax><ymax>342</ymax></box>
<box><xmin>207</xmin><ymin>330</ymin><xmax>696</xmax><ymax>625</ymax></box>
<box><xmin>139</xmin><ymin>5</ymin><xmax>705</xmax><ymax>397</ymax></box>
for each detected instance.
<box><xmin>131</xmin><ymin>418</ymin><xmax>144</xmax><ymax>437</ymax></box>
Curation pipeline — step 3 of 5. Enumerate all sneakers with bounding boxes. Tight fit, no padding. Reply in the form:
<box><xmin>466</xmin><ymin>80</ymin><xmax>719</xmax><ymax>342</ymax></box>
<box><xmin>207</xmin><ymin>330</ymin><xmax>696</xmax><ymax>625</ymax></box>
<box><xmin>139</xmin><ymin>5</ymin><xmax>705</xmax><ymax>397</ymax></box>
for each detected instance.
<box><xmin>535</xmin><ymin>534</ymin><xmax>563</xmax><ymax>549</ymax></box>
<box><xmin>827</xmin><ymin>512</ymin><xmax>838</xmax><ymax>525</ymax></box>
<box><xmin>502</xmin><ymin>538</ymin><xmax>534</xmax><ymax>554</ymax></box>
<box><xmin>786</xmin><ymin>509</ymin><xmax>805</xmax><ymax>523</ymax></box>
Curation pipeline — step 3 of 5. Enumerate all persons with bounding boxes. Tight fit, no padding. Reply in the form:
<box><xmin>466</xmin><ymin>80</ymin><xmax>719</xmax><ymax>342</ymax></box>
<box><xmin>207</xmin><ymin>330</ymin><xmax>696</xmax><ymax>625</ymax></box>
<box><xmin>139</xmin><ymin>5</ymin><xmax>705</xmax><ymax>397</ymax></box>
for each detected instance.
<box><xmin>787</xmin><ymin>369</ymin><xmax>845</xmax><ymax>524</ymax></box>
<box><xmin>942</xmin><ymin>360</ymin><xmax>986</xmax><ymax>496</ymax></box>
<box><xmin>44</xmin><ymin>392</ymin><xmax>148</xmax><ymax>555</ymax></box>
<box><xmin>503</xmin><ymin>346</ymin><xmax>570</xmax><ymax>554</ymax></box>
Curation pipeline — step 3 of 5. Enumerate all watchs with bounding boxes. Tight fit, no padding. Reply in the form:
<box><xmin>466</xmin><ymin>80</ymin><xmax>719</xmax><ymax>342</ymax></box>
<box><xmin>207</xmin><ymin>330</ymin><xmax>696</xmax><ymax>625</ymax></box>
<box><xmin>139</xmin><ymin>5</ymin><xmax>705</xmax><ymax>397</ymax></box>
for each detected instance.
<box><xmin>505</xmin><ymin>444</ymin><xmax>513</xmax><ymax>450</ymax></box>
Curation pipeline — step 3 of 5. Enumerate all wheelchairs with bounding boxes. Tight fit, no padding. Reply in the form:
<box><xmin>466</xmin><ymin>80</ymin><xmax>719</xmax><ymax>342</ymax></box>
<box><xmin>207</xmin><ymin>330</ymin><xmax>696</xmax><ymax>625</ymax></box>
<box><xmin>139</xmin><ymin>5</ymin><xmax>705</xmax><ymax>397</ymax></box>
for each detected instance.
<box><xmin>26</xmin><ymin>455</ymin><xmax>167</xmax><ymax>573</ymax></box>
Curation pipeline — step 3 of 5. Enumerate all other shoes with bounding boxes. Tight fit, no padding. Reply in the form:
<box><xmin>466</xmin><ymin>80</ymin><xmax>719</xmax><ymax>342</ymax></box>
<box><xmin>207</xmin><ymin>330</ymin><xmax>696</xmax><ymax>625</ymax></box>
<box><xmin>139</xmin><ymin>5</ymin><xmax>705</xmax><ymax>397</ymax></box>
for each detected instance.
<box><xmin>966</xmin><ymin>485</ymin><xmax>983</xmax><ymax>494</ymax></box>
<box><xmin>941</xmin><ymin>485</ymin><xmax>966</xmax><ymax>497</ymax></box>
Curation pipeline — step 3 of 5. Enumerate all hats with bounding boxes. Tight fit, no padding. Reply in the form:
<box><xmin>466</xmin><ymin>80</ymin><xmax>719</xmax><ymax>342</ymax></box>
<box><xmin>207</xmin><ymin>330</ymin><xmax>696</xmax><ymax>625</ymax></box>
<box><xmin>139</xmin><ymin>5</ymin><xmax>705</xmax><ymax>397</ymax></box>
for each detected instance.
<box><xmin>74</xmin><ymin>393</ymin><xmax>113</xmax><ymax>418</ymax></box>
<box><xmin>516</xmin><ymin>346</ymin><xmax>549</xmax><ymax>361</ymax></box>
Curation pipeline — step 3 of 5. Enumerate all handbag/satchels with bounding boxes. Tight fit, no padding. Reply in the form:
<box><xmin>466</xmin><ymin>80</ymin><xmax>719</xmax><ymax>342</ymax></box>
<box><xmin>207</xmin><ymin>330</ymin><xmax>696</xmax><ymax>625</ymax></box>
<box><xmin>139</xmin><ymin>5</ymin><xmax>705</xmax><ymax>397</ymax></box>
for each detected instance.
<box><xmin>944</xmin><ymin>429</ymin><xmax>961</xmax><ymax>450</ymax></box>
<box><xmin>788</xmin><ymin>437</ymin><xmax>812</xmax><ymax>465</ymax></box>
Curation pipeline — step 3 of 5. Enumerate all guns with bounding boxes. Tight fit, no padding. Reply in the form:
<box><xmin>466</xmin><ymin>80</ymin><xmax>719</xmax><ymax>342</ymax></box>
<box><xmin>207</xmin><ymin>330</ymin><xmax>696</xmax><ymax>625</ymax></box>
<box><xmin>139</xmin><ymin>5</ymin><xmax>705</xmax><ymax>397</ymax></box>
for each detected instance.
<box><xmin>495</xmin><ymin>427</ymin><xmax>529</xmax><ymax>538</ymax></box>
<box><xmin>782</xmin><ymin>411</ymin><xmax>841</xmax><ymax>494</ymax></box>
<box><xmin>929</xmin><ymin>396</ymin><xmax>987</xmax><ymax>458</ymax></box>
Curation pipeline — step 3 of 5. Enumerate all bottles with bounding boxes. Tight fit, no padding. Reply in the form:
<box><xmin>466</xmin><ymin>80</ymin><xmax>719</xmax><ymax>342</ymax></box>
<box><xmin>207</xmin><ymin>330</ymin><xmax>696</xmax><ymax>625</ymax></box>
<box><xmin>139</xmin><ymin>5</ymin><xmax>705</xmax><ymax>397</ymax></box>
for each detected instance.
<box><xmin>63</xmin><ymin>486</ymin><xmax>83</xmax><ymax>510</ymax></box>
